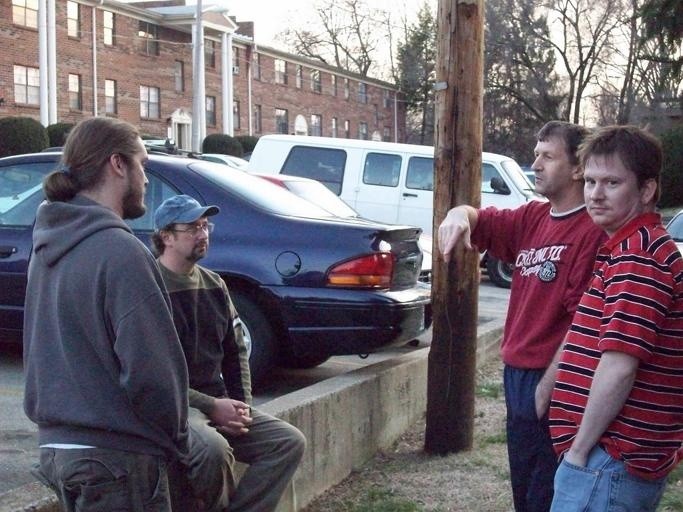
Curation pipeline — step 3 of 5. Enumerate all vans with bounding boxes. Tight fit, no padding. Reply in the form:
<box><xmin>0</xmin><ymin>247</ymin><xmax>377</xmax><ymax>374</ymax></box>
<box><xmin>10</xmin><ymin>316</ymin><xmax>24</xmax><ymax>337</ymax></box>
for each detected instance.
<box><xmin>247</xmin><ymin>132</ymin><xmax>551</xmax><ymax>290</ymax></box>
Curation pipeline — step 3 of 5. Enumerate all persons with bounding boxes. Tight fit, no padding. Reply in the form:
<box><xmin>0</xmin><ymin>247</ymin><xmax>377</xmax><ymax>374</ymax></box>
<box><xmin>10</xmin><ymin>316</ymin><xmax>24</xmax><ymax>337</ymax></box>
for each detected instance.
<box><xmin>149</xmin><ymin>192</ymin><xmax>306</xmax><ymax>511</ymax></box>
<box><xmin>436</xmin><ymin>120</ymin><xmax>609</xmax><ymax>512</ymax></box>
<box><xmin>548</xmin><ymin>117</ymin><xmax>682</xmax><ymax>512</ymax></box>
<box><xmin>20</xmin><ymin>116</ymin><xmax>190</xmax><ymax>511</ymax></box>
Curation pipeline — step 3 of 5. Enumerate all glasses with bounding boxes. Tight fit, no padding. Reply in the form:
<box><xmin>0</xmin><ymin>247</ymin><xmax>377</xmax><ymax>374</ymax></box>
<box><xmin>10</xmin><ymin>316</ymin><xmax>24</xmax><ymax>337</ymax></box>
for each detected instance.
<box><xmin>166</xmin><ymin>222</ymin><xmax>216</xmax><ymax>239</ymax></box>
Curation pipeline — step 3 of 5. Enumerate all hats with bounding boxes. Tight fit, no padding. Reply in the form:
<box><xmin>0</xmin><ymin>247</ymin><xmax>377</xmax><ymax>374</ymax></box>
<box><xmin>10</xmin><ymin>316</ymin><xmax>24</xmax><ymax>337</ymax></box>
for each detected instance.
<box><xmin>154</xmin><ymin>194</ymin><xmax>220</xmax><ymax>239</ymax></box>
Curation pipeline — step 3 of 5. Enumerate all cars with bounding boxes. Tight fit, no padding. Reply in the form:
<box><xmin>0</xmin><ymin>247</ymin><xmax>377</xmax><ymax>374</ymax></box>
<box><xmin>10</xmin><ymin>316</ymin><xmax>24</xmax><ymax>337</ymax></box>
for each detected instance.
<box><xmin>0</xmin><ymin>131</ymin><xmax>364</xmax><ymax>237</ymax></box>
<box><xmin>662</xmin><ymin>209</ymin><xmax>683</xmax><ymax>261</ymax></box>
<box><xmin>524</xmin><ymin>169</ymin><xmax>537</xmax><ymax>189</ymax></box>
<box><xmin>0</xmin><ymin>148</ymin><xmax>434</xmax><ymax>392</ymax></box>
